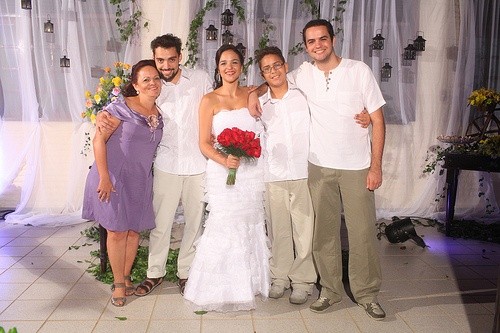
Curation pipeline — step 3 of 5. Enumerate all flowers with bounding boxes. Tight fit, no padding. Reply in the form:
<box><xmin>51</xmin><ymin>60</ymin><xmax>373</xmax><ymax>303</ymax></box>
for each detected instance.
<box><xmin>218</xmin><ymin>127</ymin><xmax>262</xmax><ymax>185</ymax></box>
<box><xmin>79</xmin><ymin>61</ymin><xmax>130</xmax><ymax>124</ymax></box>
<box><xmin>422</xmin><ymin>86</ymin><xmax>500</xmax><ymax>175</ymax></box>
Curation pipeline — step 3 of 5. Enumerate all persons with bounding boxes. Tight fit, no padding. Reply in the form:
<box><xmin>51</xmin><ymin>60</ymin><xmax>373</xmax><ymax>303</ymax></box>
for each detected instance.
<box><xmin>256</xmin><ymin>47</ymin><xmax>372</xmax><ymax>304</ymax></box>
<box><xmin>247</xmin><ymin>19</ymin><xmax>386</xmax><ymax>321</ymax></box>
<box><xmin>184</xmin><ymin>45</ymin><xmax>273</xmax><ymax>314</ymax></box>
<box><xmin>95</xmin><ymin>34</ymin><xmax>259</xmax><ymax>296</ymax></box>
<box><xmin>81</xmin><ymin>60</ymin><xmax>162</xmax><ymax>306</ymax></box>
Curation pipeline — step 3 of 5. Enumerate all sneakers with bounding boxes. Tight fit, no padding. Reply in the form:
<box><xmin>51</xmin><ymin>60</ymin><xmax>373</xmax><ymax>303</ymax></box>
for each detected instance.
<box><xmin>289</xmin><ymin>288</ymin><xmax>308</xmax><ymax>304</ymax></box>
<box><xmin>357</xmin><ymin>302</ymin><xmax>386</xmax><ymax>321</ymax></box>
<box><xmin>309</xmin><ymin>296</ymin><xmax>343</xmax><ymax>313</ymax></box>
<box><xmin>269</xmin><ymin>284</ymin><xmax>288</xmax><ymax>298</ymax></box>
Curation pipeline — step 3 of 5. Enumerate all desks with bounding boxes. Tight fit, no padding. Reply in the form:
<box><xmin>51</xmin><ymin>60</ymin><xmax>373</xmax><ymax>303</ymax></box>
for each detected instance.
<box><xmin>443</xmin><ymin>150</ymin><xmax>500</xmax><ymax>237</ymax></box>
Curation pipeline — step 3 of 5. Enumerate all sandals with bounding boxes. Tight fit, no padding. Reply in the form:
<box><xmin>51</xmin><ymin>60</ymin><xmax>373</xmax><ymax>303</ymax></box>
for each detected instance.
<box><xmin>134</xmin><ymin>277</ymin><xmax>163</xmax><ymax>297</ymax></box>
<box><xmin>110</xmin><ymin>283</ymin><xmax>127</xmax><ymax>307</ymax></box>
<box><xmin>177</xmin><ymin>279</ymin><xmax>188</xmax><ymax>296</ymax></box>
<box><xmin>124</xmin><ymin>275</ymin><xmax>136</xmax><ymax>296</ymax></box>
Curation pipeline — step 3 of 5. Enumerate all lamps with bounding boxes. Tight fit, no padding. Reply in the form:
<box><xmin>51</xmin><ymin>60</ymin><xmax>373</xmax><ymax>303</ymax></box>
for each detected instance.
<box><xmin>222</xmin><ymin>30</ymin><xmax>233</xmax><ymax>44</ymax></box>
<box><xmin>384</xmin><ymin>217</ymin><xmax>427</xmax><ymax>248</ymax></box>
<box><xmin>44</xmin><ymin>20</ymin><xmax>53</xmax><ymax>32</ymax></box>
<box><xmin>221</xmin><ymin>9</ymin><xmax>234</xmax><ymax>26</ymax></box>
<box><xmin>372</xmin><ymin>29</ymin><xmax>385</xmax><ymax>50</ymax></box>
<box><xmin>205</xmin><ymin>25</ymin><xmax>218</xmax><ymax>40</ymax></box>
<box><xmin>380</xmin><ymin>62</ymin><xmax>392</xmax><ymax>79</ymax></box>
<box><xmin>59</xmin><ymin>55</ymin><xmax>70</xmax><ymax>68</ymax></box>
<box><xmin>21</xmin><ymin>0</ymin><xmax>31</xmax><ymax>9</ymax></box>
<box><xmin>403</xmin><ymin>44</ymin><xmax>417</xmax><ymax>60</ymax></box>
<box><xmin>413</xmin><ymin>36</ymin><xmax>426</xmax><ymax>52</ymax></box>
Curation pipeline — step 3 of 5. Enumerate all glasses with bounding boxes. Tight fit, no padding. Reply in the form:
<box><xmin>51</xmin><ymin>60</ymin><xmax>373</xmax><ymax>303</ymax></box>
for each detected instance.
<box><xmin>262</xmin><ymin>63</ymin><xmax>284</xmax><ymax>74</ymax></box>
